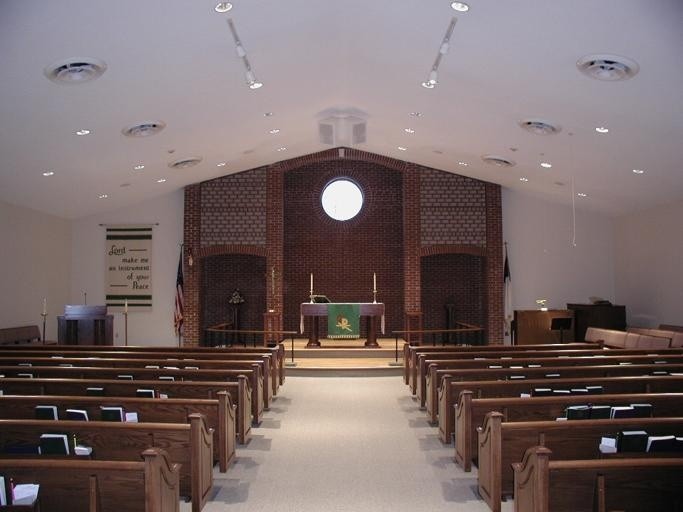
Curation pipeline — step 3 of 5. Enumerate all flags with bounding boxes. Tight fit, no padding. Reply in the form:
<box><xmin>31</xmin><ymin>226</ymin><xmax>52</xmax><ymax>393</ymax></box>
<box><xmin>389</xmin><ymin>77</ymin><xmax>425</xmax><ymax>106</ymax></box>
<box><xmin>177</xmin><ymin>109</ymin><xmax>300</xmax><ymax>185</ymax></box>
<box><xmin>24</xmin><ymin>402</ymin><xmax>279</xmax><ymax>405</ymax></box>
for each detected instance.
<box><xmin>502</xmin><ymin>252</ymin><xmax>514</xmax><ymax>338</ymax></box>
<box><xmin>173</xmin><ymin>247</ymin><xmax>184</xmax><ymax>336</ymax></box>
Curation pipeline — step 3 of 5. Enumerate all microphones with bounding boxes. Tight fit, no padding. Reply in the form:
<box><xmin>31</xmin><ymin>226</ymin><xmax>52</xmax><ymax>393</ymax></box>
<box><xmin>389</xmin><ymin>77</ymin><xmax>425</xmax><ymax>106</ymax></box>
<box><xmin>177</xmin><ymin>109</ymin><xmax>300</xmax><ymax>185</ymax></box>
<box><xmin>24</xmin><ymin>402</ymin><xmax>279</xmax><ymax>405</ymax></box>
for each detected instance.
<box><xmin>84</xmin><ymin>292</ymin><xmax>87</xmax><ymax>305</ymax></box>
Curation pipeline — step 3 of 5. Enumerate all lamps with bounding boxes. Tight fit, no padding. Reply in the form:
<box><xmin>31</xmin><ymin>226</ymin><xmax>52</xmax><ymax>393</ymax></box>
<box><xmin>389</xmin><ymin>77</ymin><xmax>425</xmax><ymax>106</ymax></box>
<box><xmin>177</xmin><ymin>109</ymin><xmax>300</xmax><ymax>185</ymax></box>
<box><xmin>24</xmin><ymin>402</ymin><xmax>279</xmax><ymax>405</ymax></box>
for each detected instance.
<box><xmin>245</xmin><ymin>63</ymin><xmax>264</xmax><ymax>90</ymax></box>
<box><xmin>421</xmin><ymin>64</ymin><xmax>440</xmax><ymax>90</ymax></box>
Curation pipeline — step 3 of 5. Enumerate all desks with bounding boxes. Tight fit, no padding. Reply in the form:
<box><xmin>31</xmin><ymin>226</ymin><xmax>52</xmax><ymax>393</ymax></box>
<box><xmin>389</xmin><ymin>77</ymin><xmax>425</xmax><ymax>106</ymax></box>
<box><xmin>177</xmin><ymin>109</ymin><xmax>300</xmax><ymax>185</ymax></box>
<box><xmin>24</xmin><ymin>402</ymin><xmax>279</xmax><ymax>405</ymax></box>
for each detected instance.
<box><xmin>300</xmin><ymin>303</ymin><xmax>385</xmax><ymax>348</ymax></box>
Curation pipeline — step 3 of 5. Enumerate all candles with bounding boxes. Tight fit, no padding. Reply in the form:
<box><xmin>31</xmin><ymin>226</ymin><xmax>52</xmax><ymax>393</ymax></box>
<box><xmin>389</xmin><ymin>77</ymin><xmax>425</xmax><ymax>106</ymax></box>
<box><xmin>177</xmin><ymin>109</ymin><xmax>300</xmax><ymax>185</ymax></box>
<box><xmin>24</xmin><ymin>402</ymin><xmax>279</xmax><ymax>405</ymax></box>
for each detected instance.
<box><xmin>372</xmin><ymin>273</ymin><xmax>378</xmax><ymax>291</ymax></box>
<box><xmin>43</xmin><ymin>296</ymin><xmax>46</xmax><ymax>313</ymax></box>
<box><xmin>125</xmin><ymin>298</ymin><xmax>127</xmax><ymax>312</ymax></box>
<box><xmin>309</xmin><ymin>272</ymin><xmax>316</xmax><ymax>291</ymax></box>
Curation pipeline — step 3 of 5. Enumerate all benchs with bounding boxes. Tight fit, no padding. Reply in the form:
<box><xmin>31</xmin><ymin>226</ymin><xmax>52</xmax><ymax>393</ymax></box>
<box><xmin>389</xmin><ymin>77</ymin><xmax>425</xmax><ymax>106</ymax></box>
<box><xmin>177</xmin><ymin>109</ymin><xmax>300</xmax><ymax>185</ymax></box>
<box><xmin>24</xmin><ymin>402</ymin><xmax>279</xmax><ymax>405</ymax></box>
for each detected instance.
<box><xmin>0</xmin><ymin>324</ymin><xmax>285</xmax><ymax>512</ymax></box>
<box><xmin>400</xmin><ymin>324</ymin><xmax>683</xmax><ymax>511</ymax></box>
